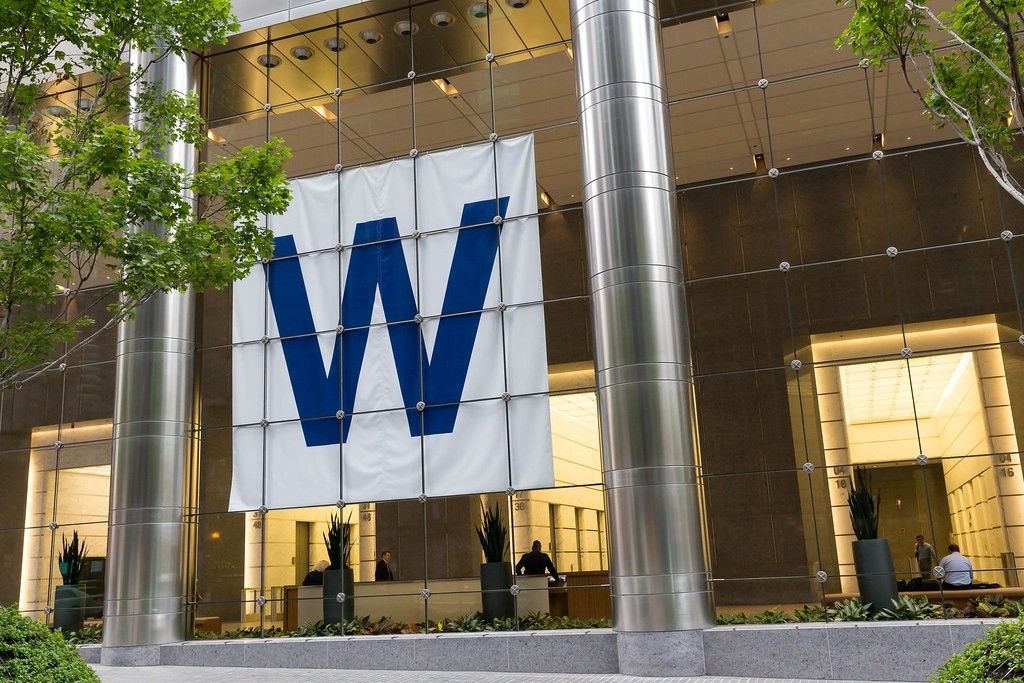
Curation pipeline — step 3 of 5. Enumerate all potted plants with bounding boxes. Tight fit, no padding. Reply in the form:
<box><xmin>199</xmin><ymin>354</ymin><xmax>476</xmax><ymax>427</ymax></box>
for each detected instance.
<box><xmin>51</xmin><ymin>532</ymin><xmax>87</xmax><ymax>636</ymax></box>
<box><xmin>323</xmin><ymin>511</ymin><xmax>355</xmax><ymax>631</ymax></box>
<box><xmin>845</xmin><ymin>465</ymin><xmax>903</xmax><ymax>612</ymax></box>
<box><xmin>471</xmin><ymin>503</ymin><xmax>516</xmax><ymax>624</ymax></box>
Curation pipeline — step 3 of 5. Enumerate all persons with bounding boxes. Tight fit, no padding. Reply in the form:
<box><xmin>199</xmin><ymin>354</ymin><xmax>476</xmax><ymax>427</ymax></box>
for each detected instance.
<box><xmin>516</xmin><ymin>540</ymin><xmax>563</xmax><ymax>581</ymax></box>
<box><xmin>303</xmin><ymin>560</ymin><xmax>329</xmax><ymax>585</ymax></box>
<box><xmin>374</xmin><ymin>552</ymin><xmax>393</xmax><ymax>582</ymax></box>
<box><xmin>937</xmin><ymin>544</ymin><xmax>974</xmax><ymax>590</ymax></box>
<box><xmin>913</xmin><ymin>535</ymin><xmax>938</xmax><ymax>580</ymax></box>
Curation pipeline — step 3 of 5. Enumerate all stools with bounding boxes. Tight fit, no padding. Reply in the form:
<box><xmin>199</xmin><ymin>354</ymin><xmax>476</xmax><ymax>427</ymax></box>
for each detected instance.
<box><xmin>193</xmin><ymin>617</ymin><xmax>222</xmax><ymax>635</ymax></box>
